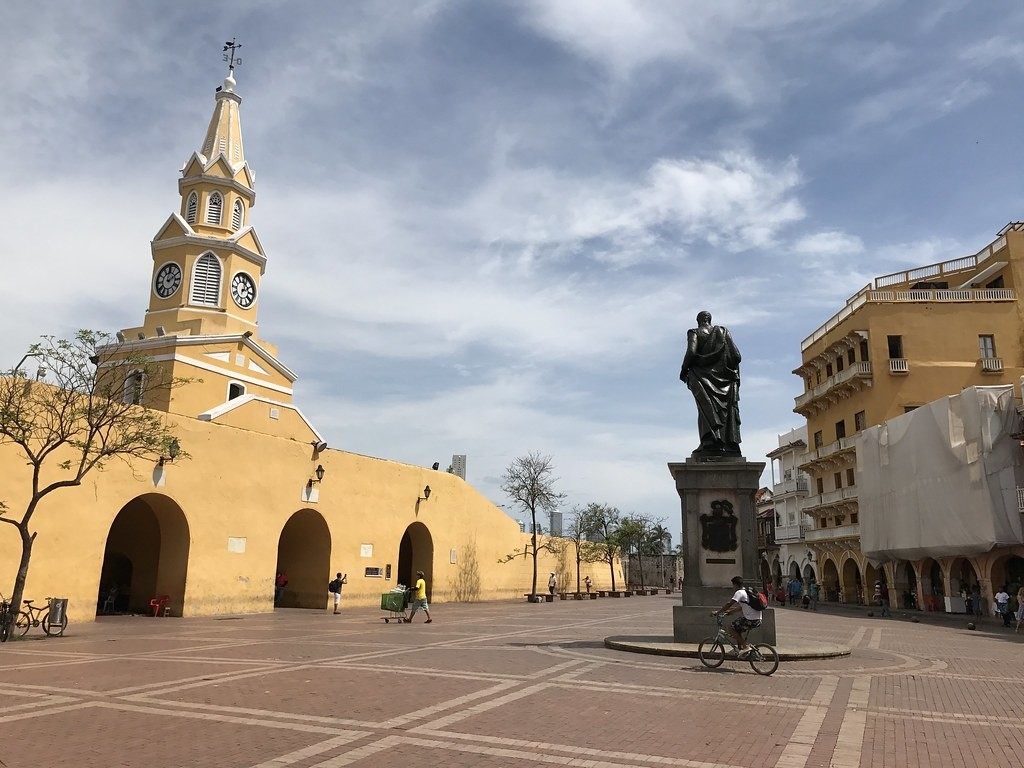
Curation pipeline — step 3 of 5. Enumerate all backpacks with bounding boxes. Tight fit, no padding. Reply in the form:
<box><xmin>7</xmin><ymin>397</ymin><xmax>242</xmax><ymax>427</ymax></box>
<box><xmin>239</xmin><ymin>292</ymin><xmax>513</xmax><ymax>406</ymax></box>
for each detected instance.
<box><xmin>329</xmin><ymin>579</ymin><xmax>338</xmax><ymax>592</ymax></box>
<box><xmin>734</xmin><ymin>586</ymin><xmax>767</xmax><ymax>611</ymax></box>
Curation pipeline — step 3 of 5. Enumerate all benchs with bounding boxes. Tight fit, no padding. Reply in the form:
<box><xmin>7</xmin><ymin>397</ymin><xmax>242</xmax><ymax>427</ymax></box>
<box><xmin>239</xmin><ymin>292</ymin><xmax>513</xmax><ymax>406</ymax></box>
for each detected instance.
<box><xmin>556</xmin><ymin>587</ymin><xmax>673</xmax><ymax>600</ymax></box>
<box><xmin>523</xmin><ymin>594</ymin><xmax>557</xmax><ymax>602</ymax></box>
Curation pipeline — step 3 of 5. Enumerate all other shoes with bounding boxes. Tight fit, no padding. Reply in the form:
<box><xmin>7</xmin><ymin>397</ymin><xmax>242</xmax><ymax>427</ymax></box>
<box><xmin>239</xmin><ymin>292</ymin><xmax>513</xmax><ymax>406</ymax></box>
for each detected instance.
<box><xmin>424</xmin><ymin>620</ymin><xmax>432</xmax><ymax>623</ymax></box>
<box><xmin>334</xmin><ymin>612</ymin><xmax>341</xmax><ymax>614</ymax></box>
<box><xmin>729</xmin><ymin>650</ymin><xmax>738</xmax><ymax>655</ymax></box>
<box><xmin>738</xmin><ymin>645</ymin><xmax>752</xmax><ymax>657</ymax></box>
<box><xmin>405</xmin><ymin>619</ymin><xmax>411</xmax><ymax>623</ymax></box>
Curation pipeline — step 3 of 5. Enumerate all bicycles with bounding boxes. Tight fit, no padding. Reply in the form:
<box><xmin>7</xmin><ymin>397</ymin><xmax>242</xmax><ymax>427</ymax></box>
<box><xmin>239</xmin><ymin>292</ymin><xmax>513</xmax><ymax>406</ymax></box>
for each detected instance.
<box><xmin>696</xmin><ymin>610</ymin><xmax>780</xmax><ymax>676</ymax></box>
<box><xmin>0</xmin><ymin>592</ymin><xmax>68</xmax><ymax>643</ymax></box>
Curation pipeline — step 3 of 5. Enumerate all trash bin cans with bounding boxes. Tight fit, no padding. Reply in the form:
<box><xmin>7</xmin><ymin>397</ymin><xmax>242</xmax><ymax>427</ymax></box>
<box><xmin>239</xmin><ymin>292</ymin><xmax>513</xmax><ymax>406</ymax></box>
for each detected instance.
<box><xmin>48</xmin><ymin>598</ymin><xmax>69</xmax><ymax>623</ymax></box>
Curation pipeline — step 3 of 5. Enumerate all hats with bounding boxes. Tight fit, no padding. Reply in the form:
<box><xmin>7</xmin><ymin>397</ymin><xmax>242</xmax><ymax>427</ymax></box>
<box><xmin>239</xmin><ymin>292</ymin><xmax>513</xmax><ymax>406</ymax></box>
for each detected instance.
<box><xmin>417</xmin><ymin>571</ymin><xmax>424</xmax><ymax>575</ymax></box>
<box><xmin>816</xmin><ymin>582</ymin><xmax>821</xmax><ymax>585</ymax></box>
<box><xmin>551</xmin><ymin>572</ymin><xmax>555</xmax><ymax>574</ymax></box>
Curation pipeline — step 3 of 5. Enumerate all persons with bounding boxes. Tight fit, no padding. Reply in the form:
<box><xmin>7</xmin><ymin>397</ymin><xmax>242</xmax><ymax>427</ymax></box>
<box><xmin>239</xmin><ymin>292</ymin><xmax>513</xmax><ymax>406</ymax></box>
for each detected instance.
<box><xmin>333</xmin><ymin>573</ymin><xmax>347</xmax><ymax>614</ymax></box>
<box><xmin>669</xmin><ymin>575</ymin><xmax>675</xmax><ymax>592</ymax></box>
<box><xmin>403</xmin><ymin>571</ymin><xmax>432</xmax><ymax>623</ymax></box>
<box><xmin>275</xmin><ymin>572</ymin><xmax>288</xmax><ymax>608</ymax></box>
<box><xmin>764</xmin><ymin>576</ymin><xmax>819</xmax><ymax>612</ymax></box>
<box><xmin>875</xmin><ymin>581</ymin><xmax>1024</xmax><ymax>632</ymax></box>
<box><xmin>585</xmin><ymin>576</ymin><xmax>592</xmax><ymax>594</ymax></box>
<box><xmin>710</xmin><ymin>576</ymin><xmax>763</xmax><ymax>657</ymax></box>
<box><xmin>548</xmin><ymin>572</ymin><xmax>557</xmax><ymax>594</ymax></box>
<box><xmin>680</xmin><ymin>311</ymin><xmax>741</xmax><ymax>453</ymax></box>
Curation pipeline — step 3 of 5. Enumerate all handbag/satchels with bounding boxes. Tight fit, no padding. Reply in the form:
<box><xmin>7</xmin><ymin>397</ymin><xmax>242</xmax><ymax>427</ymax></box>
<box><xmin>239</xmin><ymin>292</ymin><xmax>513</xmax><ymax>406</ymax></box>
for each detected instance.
<box><xmin>781</xmin><ymin>602</ymin><xmax>785</xmax><ymax>606</ymax></box>
<box><xmin>589</xmin><ymin>583</ymin><xmax>592</xmax><ymax>586</ymax></box>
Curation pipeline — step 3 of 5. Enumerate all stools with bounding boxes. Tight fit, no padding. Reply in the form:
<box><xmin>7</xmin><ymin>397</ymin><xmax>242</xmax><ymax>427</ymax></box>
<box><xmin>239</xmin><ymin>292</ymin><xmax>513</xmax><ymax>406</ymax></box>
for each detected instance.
<box><xmin>163</xmin><ymin>606</ymin><xmax>170</xmax><ymax>617</ymax></box>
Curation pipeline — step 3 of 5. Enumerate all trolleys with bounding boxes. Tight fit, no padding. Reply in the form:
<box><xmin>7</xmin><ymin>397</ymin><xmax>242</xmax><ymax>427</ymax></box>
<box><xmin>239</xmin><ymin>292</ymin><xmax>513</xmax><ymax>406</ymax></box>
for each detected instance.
<box><xmin>380</xmin><ymin>587</ymin><xmax>414</xmax><ymax>623</ymax></box>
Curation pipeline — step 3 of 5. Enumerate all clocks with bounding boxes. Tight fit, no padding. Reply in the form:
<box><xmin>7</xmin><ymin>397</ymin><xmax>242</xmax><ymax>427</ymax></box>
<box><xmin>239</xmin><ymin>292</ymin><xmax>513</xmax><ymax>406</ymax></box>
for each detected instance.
<box><xmin>231</xmin><ymin>271</ymin><xmax>254</xmax><ymax>307</ymax></box>
<box><xmin>155</xmin><ymin>262</ymin><xmax>181</xmax><ymax>297</ymax></box>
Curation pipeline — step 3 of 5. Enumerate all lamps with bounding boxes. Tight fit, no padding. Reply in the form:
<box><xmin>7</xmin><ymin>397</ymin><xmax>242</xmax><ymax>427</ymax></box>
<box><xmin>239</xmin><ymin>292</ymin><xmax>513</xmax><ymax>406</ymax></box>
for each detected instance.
<box><xmin>417</xmin><ymin>485</ymin><xmax>431</xmax><ymax>502</ymax></box>
<box><xmin>308</xmin><ymin>463</ymin><xmax>325</xmax><ymax>487</ymax></box>
<box><xmin>310</xmin><ymin>438</ymin><xmax>327</xmax><ymax>452</ymax></box>
<box><xmin>158</xmin><ymin>436</ymin><xmax>180</xmax><ymax>466</ymax></box>
